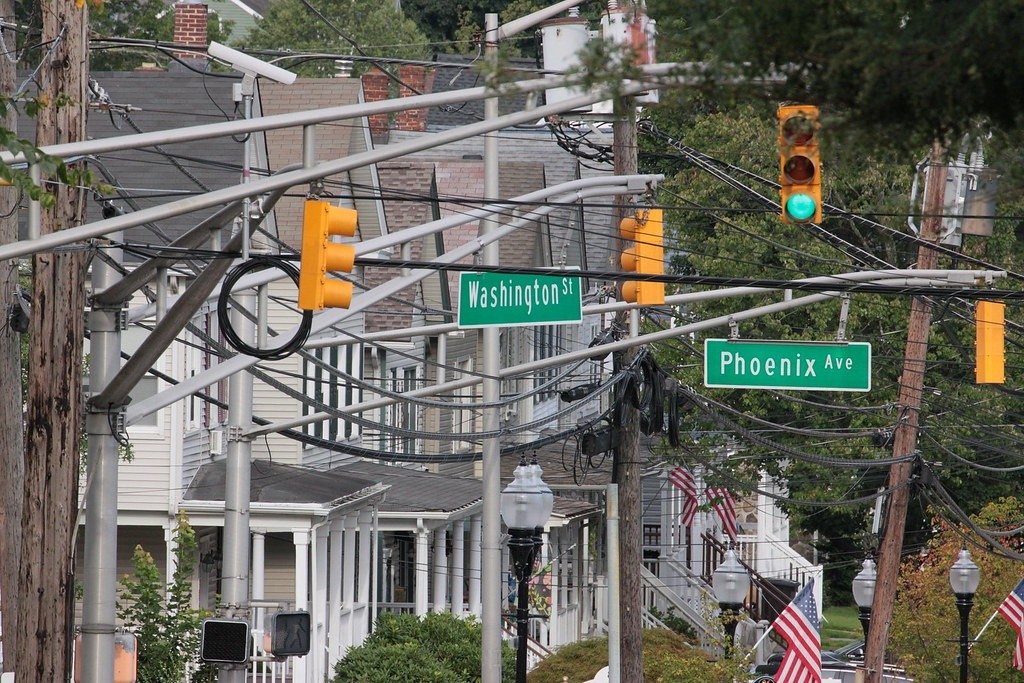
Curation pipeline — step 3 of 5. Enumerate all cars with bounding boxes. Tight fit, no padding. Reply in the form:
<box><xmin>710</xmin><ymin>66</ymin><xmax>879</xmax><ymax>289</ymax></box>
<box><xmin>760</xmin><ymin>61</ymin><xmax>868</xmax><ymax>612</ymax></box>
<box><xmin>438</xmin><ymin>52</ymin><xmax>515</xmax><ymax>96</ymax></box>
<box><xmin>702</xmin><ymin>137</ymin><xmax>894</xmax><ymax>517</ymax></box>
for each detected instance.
<box><xmin>752</xmin><ymin>641</ymin><xmax>918</xmax><ymax>683</ymax></box>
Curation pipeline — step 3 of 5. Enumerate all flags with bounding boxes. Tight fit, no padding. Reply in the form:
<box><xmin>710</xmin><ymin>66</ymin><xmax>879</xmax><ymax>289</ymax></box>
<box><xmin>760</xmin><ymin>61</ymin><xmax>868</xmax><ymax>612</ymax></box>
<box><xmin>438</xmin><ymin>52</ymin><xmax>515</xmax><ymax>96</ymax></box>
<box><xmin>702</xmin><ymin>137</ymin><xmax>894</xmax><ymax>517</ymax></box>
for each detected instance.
<box><xmin>668</xmin><ymin>459</ymin><xmax>698</xmax><ymax>528</ymax></box>
<box><xmin>706</xmin><ymin>471</ymin><xmax>736</xmax><ymax>544</ymax></box>
<box><xmin>997</xmin><ymin>577</ymin><xmax>1024</xmax><ymax>672</ymax></box>
<box><xmin>772</xmin><ymin>578</ymin><xmax>821</xmax><ymax>683</ymax></box>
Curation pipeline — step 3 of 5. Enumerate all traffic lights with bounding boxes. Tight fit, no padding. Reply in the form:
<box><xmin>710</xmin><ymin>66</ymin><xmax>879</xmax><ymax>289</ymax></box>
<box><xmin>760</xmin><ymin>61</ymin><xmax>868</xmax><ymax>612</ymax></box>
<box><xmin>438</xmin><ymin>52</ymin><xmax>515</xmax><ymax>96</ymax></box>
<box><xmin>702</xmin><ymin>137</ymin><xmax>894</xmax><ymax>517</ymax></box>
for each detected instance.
<box><xmin>265</xmin><ymin>611</ymin><xmax>311</xmax><ymax>656</ymax></box>
<box><xmin>778</xmin><ymin>105</ymin><xmax>823</xmax><ymax>225</ymax></box>
<box><xmin>295</xmin><ymin>199</ymin><xmax>358</xmax><ymax>310</ymax></box>
<box><xmin>202</xmin><ymin>619</ymin><xmax>251</xmax><ymax>663</ymax></box>
<box><xmin>619</xmin><ymin>210</ymin><xmax>665</xmax><ymax>305</ymax></box>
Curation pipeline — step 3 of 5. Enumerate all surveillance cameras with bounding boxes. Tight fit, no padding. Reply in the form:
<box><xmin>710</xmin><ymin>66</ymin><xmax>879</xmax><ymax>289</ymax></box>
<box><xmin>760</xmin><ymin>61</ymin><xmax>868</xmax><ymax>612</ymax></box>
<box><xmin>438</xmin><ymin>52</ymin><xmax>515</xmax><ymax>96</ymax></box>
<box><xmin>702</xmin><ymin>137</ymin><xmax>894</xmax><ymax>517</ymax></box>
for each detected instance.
<box><xmin>206</xmin><ymin>41</ymin><xmax>297</xmax><ymax>85</ymax></box>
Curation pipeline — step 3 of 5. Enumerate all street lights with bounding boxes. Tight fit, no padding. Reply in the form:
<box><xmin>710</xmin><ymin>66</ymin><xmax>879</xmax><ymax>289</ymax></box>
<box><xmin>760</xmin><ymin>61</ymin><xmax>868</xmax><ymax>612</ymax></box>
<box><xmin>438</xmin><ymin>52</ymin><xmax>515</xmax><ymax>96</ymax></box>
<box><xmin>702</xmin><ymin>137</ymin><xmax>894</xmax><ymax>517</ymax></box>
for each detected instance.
<box><xmin>851</xmin><ymin>551</ymin><xmax>876</xmax><ymax>648</ymax></box>
<box><xmin>949</xmin><ymin>540</ymin><xmax>981</xmax><ymax>683</ymax></box>
<box><xmin>711</xmin><ymin>538</ymin><xmax>750</xmax><ymax>660</ymax></box>
<box><xmin>501</xmin><ymin>451</ymin><xmax>554</xmax><ymax>683</ymax></box>
